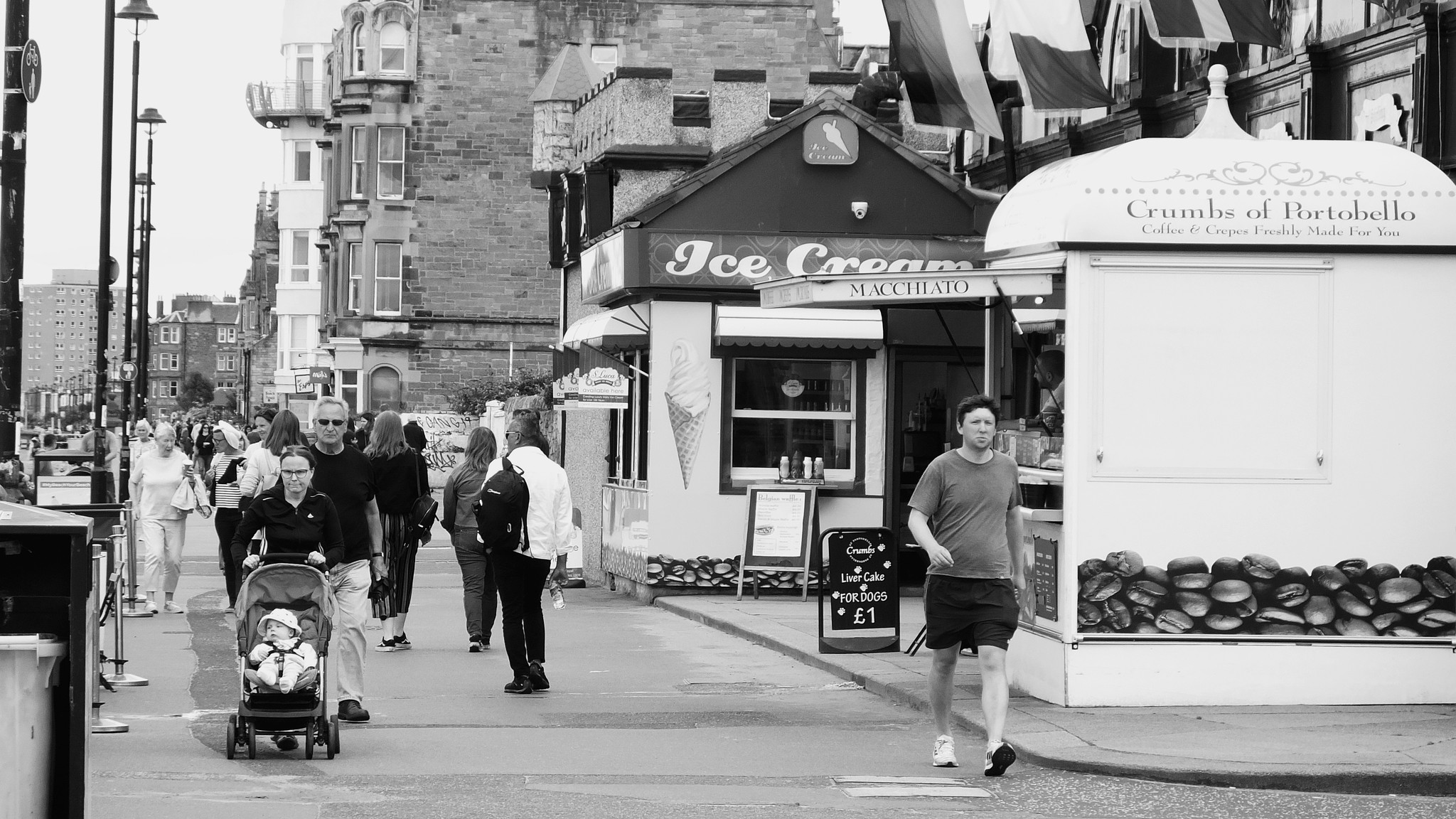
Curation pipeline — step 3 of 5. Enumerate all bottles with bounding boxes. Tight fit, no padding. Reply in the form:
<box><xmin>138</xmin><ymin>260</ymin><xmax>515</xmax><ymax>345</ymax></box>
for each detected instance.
<box><xmin>548</xmin><ymin>580</ymin><xmax>566</xmax><ymax>610</ymax></box>
<box><xmin>791</xmin><ymin>450</ymin><xmax>802</xmax><ymax>479</ymax></box>
<box><xmin>802</xmin><ymin>457</ymin><xmax>813</xmax><ymax>479</ymax></box>
<box><xmin>779</xmin><ymin>456</ymin><xmax>790</xmax><ymax>478</ymax></box>
<box><xmin>813</xmin><ymin>457</ymin><xmax>824</xmax><ymax>479</ymax></box>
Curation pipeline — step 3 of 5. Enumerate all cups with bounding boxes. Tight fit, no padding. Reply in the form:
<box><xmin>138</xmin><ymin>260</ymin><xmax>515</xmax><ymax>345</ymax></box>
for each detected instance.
<box><xmin>1042</xmin><ymin>412</ymin><xmax>1058</xmax><ymax>432</ymax></box>
<box><xmin>182</xmin><ymin>459</ymin><xmax>193</xmax><ymax>476</ymax></box>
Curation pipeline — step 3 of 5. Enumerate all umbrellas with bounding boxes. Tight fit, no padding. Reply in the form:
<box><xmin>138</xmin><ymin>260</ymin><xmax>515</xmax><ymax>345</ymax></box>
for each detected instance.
<box><xmin>367</xmin><ymin>555</ymin><xmax>394</xmax><ymax>621</ymax></box>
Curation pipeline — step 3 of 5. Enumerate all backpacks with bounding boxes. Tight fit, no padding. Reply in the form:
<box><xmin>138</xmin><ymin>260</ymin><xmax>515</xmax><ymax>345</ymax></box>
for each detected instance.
<box><xmin>471</xmin><ymin>456</ymin><xmax>530</xmax><ymax>554</ymax></box>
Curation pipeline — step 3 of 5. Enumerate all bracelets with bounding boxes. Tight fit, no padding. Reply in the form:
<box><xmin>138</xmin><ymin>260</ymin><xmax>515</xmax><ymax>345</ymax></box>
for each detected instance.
<box><xmin>189</xmin><ymin>482</ymin><xmax>194</xmax><ymax>483</ymax></box>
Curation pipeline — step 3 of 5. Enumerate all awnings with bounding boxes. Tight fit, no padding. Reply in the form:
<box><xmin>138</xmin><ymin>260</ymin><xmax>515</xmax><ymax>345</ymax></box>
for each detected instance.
<box><xmin>563</xmin><ymin>303</ymin><xmax>651</xmax><ymax>352</ymax></box>
<box><xmin>712</xmin><ymin>304</ymin><xmax>885</xmax><ymax>351</ymax></box>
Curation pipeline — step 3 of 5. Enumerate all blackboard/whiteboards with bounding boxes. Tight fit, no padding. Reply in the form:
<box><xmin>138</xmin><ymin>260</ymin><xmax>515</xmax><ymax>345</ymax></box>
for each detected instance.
<box><xmin>831</xmin><ymin>529</ymin><xmax>898</xmax><ymax>630</ymax></box>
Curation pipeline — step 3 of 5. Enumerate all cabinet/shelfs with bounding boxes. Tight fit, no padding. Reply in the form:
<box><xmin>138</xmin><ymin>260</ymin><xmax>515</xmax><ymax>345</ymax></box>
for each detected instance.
<box><xmin>792</xmin><ymin>378</ymin><xmax>845</xmax><ymax>469</ymax></box>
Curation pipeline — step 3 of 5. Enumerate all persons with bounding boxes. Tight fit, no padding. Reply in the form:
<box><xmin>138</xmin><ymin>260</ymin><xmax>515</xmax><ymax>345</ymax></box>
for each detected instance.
<box><xmin>128</xmin><ymin>421</ymin><xmax>195</xmax><ymax>614</ymax></box>
<box><xmin>477</xmin><ymin>408</ymin><xmax>573</xmax><ymax>694</ymax></box>
<box><xmin>907</xmin><ymin>394</ymin><xmax>1026</xmax><ymax>777</ymax></box>
<box><xmin>229</xmin><ymin>449</ymin><xmax>345</xmax><ymax>750</ymax></box>
<box><xmin>365</xmin><ymin>410</ymin><xmax>431</xmax><ymax>652</ymax></box>
<box><xmin>439</xmin><ymin>426</ymin><xmax>498</xmax><ymax>652</ymax></box>
<box><xmin>276</xmin><ymin>396</ymin><xmax>388</xmax><ymax>721</ymax></box>
<box><xmin>30</xmin><ymin>407</ymin><xmax>427</xmax><ymax>613</ymax></box>
<box><xmin>247</xmin><ymin>608</ymin><xmax>318</xmax><ymax>694</ymax></box>
<box><xmin>996</xmin><ymin>350</ymin><xmax>1065</xmax><ymax>432</ymax></box>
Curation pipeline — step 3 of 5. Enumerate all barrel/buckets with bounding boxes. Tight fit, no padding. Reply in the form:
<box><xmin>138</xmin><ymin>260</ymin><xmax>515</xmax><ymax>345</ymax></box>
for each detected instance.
<box><xmin>1019</xmin><ymin>483</ymin><xmax>1048</xmax><ymax>509</ymax></box>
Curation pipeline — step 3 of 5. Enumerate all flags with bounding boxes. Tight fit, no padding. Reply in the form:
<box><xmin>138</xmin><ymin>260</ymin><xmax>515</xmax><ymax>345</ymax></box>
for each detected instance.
<box><xmin>563</xmin><ymin>344</ymin><xmax>580</xmax><ymax>407</ymax></box>
<box><xmin>578</xmin><ymin>340</ymin><xmax>630</xmax><ymax>409</ymax></box>
<box><xmin>552</xmin><ymin>346</ymin><xmax>578</xmax><ymax>411</ymax></box>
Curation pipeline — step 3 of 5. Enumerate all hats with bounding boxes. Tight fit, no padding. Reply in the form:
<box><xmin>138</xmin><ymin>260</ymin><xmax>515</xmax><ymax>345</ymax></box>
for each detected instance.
<box><xmin>257</xmin><ymin>608</ymin><xmax>302</xmax><ymax>637</ymax></box>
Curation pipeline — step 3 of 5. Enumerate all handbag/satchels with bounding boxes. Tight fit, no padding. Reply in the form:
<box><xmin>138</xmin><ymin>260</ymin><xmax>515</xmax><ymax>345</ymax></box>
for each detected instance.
<box><xmin>409</xmin><ymin>490</ymin><xmax>439</xmax><ymax>548</ymax></box>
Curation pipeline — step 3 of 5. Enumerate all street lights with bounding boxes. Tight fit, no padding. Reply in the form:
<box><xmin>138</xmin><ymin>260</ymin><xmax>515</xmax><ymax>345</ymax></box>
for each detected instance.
<box><xmin>44</xmin><ymin>356</ymin><xmax>118</xmax><ymax>405</ymax></box>
<box><xmin>116</xmin><ymin>0</ymin><xmax>166</xmax><ymax>521</ymax></box>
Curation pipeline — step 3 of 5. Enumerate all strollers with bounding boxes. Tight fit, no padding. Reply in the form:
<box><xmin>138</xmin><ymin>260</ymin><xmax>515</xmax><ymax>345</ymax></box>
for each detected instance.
<box><xmin>226</xmin><ymin>552</ymin><xmax>340</xmax><ymax>760</ymax></box>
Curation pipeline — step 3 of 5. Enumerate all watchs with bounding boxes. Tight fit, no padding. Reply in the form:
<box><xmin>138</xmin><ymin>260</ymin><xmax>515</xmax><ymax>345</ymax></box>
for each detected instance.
<box><xmin>372</xmin><ymin>551</ymin><xmax>384</xmax><ymax>558</ymax></box>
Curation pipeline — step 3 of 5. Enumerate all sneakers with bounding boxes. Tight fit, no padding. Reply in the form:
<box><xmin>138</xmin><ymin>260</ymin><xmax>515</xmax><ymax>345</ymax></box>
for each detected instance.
<box><xmin>276</xmin><ymin>733</ymin><xmax>299</xmax><ymax>750</ymax></box>
<box><xmin>932</xmin><ymin>734</ymin><xmax>959</xmax><ymax>768</ymax></box>
<box><xmin>393</xmin><ymin>631</ymin><xmax>412</xmax><ymax>649</ymax></box>
<box><xmin>164</xmin><ymin>601</ymin><xmax>184</xmax><ymax>613</ymax></box>
<box><xmin>338</xmin><ymin>700</ymin><xmax>370</xmax><ymax>721</ymax></box>
<box><xmin>983</xmin><ymin>740</ymin><xmax>1017</xmax><ymax>776</ymax></box>
<box><xmin>375</xmin><ymin>636</ymin><xmax>395</xmax><ymax>652</ymax></box>
<box><xmin>504</xmin><ymin>675</ymin><xmax>532</xmax><ymax>694</ymax></box>
<box><xmin>480</xmin><ymin>636</ymin><xmax>491</xmax><ymax>649</ymax></box>
<box><xmin>529</xmin><ymin>660</ymin><xmax>550</xmax><ymax>690</ymax></box>
<box><xmin>225</xmin><ymin>606</ymin><xmax>234</xmax><ymax>613</ymax></box>
<box><xmin>469</xmin><ymin>635</ymin><xmax>483</xmax><ymax>652</ymax></box>
<box><xmin>144</xmin><ymin>600</ymin><xmax>158</xmax><ymax>614</ymax></box>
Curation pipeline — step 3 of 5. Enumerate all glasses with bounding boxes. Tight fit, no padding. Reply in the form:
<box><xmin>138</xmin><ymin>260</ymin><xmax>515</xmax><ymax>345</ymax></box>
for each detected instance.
<box><xmin>212</xmin><ymin>438</ymin><xmax>226</xmax><ymax>444</ymax></box>
<box><xmin>505</xmin><ymin>430</ymin><xmax>524</xmax><ymax>439</ymax></box>
<box><xmin>317</xmin><ymin>418</ymin><xmax>346</xmax><ymax>426</ymax></box>
<box><xmin>203</xmin><ymin>426</ymin><xmax>208</xmax><ymax>429</ymax></box>
<box><xmin>281</xmin><ymin>469</ymin><xmax>309</xmax><ymax>479</ymax></box>
